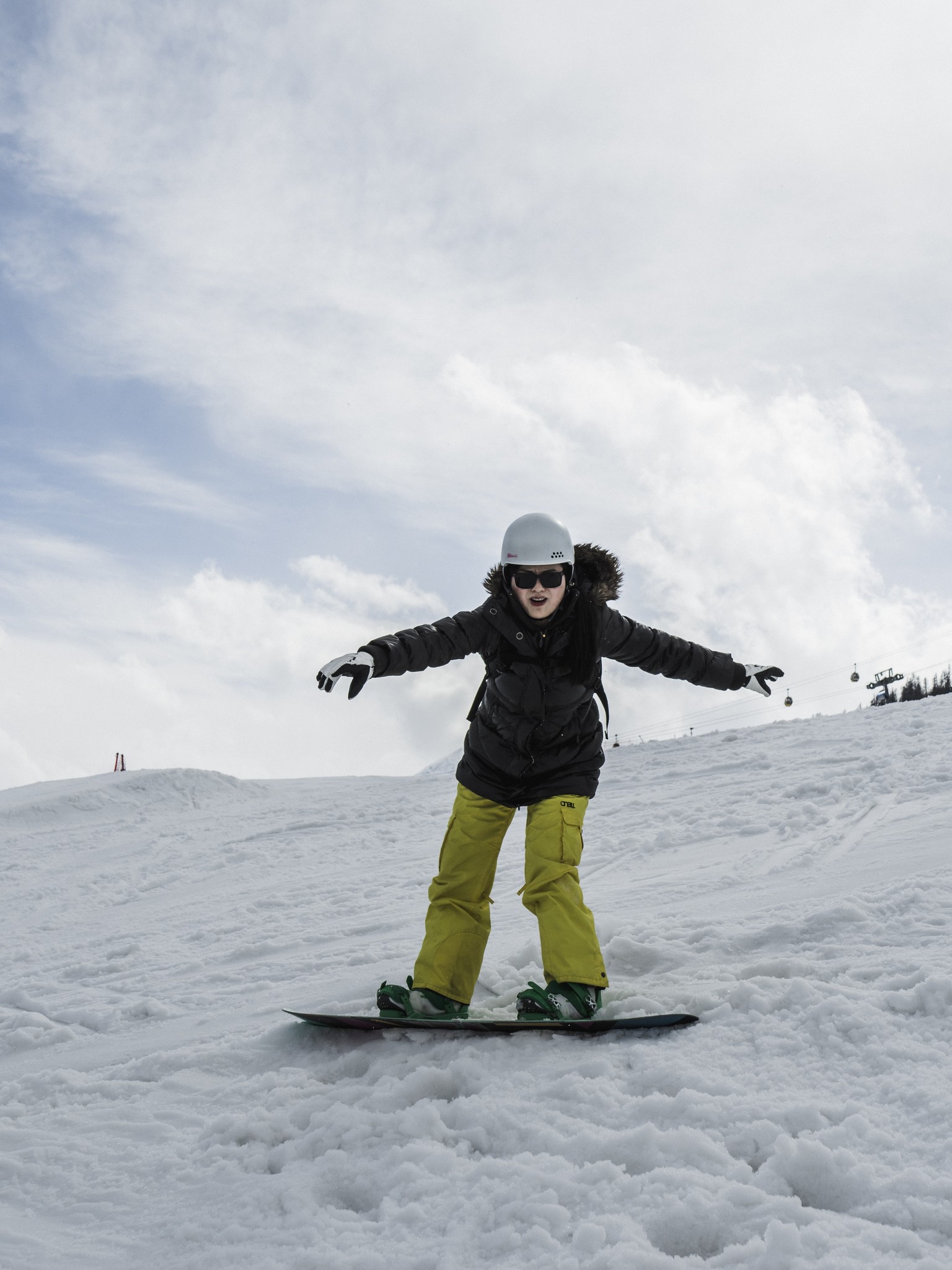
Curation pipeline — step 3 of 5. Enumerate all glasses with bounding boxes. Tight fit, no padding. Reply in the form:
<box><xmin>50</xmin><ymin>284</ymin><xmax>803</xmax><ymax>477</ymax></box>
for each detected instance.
<box><xmin>511</xmin><ymin>570</ymin><xmax>565</xmax><ymax>589</ymax></box>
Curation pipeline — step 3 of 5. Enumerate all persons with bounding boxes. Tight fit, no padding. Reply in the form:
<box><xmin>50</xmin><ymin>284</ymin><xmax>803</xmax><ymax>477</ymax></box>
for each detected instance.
<box><xmin>317</xmin><ymin>513</ymin><xmax>784</xmax><ymax>1019</ymax></box>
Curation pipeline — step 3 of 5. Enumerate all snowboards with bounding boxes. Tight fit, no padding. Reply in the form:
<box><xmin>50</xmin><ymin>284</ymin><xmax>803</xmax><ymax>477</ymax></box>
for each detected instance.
<box><xmin>281</xmin><ymin>1006</ymin><xmax>700</xmax><ymax>1028</ymax></box>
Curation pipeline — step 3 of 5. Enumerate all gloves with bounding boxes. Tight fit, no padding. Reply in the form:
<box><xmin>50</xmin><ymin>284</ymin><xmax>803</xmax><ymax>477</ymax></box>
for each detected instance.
<box><xmin>316</xmin><ymin>651</ymin><xmax>375</xmax><ymax>700</ymax></box>
<box><xmin>743</xmin><ymin>664</ymin><xmax>784</xmax><ymax>697</ymax></box>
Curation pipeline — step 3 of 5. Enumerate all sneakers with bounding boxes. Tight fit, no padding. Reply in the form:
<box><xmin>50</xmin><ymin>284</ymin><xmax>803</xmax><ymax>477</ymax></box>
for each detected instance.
<box><xmin>376</xmin><ymin>975</ymin><xmax>469</xmax><ymax>1019</ymax></box>
<box><xmin>517</xmin><ymin>981</ymin><xmax>603</xmax><ymax>1021</ymax></box>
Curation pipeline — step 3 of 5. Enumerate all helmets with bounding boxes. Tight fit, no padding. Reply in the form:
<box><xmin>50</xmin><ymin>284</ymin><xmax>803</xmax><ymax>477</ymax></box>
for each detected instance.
<box><xmin>500</xmin><ymin>513</ymin><xmax>575</xmax><ymax>584</ymax></box>
